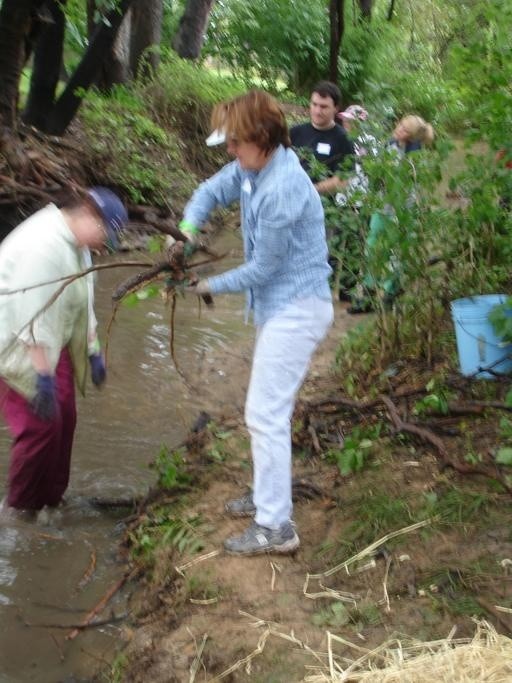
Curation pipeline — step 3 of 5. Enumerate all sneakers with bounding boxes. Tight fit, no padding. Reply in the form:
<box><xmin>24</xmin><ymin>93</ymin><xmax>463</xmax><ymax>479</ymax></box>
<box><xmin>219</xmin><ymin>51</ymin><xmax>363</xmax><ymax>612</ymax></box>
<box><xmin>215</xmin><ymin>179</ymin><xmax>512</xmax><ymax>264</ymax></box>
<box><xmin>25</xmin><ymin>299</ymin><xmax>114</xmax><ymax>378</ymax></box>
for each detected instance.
<box><xmin>225</xmin><ymin>491</ymin><xmax>256</xmax><ymax>518</ymax></box>
<box><xmin>221</xmin><ymin>520</ymin><xmax>301</xmax><ymax>558</ymax></box>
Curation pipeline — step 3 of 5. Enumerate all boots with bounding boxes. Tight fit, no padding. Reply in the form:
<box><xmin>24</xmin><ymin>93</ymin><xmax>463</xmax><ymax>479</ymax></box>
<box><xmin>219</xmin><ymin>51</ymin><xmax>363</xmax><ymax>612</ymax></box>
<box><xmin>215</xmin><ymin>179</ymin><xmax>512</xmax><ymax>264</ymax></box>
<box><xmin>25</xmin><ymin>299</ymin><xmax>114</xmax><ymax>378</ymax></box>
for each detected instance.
<box><xmin>339</xmin><ymin>287</ymin><xmax>393</xmax><ymax>315</ymax></box>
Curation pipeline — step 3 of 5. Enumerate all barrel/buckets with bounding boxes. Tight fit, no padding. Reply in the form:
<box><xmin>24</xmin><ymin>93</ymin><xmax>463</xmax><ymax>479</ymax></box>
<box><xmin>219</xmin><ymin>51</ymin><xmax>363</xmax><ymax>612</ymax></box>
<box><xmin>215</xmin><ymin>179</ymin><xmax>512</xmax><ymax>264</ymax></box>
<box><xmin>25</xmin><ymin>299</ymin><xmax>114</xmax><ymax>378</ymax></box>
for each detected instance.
<box><xmin>449</xmin><ymin>296</ymin><xmax>511</xmax><ymax>379</ymax></box>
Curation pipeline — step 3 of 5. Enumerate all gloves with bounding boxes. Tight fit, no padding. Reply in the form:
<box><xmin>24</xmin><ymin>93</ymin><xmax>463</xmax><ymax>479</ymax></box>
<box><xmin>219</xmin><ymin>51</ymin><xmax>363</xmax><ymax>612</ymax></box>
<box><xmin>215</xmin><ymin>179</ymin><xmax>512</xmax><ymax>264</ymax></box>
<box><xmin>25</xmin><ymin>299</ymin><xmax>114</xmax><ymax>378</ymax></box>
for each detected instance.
<box><xmin>88</xmin><ymin>354</ymin><xmax>105</xmax><ymax>389</ymax></box>
<box><xmin>32</xmin><ymin>373</ymin><xmax>62</xmax><ymax>425</ymax></box>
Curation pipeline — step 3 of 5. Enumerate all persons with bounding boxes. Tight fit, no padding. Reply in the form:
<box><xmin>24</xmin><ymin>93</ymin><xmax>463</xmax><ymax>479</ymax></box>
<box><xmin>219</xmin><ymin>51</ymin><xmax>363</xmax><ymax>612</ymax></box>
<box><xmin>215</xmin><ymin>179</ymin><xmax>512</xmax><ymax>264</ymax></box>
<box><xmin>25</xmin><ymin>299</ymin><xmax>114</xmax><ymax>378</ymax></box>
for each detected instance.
<box><xmin>1</xmin><ymin>187</ymin><xmax>132</xmax><ymax>526</ymax></box>
<box><xmin>166</xmin><ymin>91</ymin><xmax>338</xmax><ymax>558</ymax></box>
<box><xmin>284</xmin><ymin>80</ymin><xmax>436</xmax><ymax>314</ymax></box>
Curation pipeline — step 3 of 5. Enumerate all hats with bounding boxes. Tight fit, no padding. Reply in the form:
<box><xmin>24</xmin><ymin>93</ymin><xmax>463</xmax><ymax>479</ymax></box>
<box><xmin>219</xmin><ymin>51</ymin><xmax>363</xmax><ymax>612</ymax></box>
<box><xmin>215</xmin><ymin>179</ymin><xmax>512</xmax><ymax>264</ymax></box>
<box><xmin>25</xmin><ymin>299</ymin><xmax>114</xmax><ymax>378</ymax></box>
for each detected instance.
<box><xmin>84</xmin><ymin>187</ymin><xmax>129</xmax><ymax>251</ymax></box>
<box><xmin>335</xmin><ymin>104</ymin><xmax>368</xmax><ymax>123</ymax></box>
<box><xmin>206</xmin><ymin>123</ymin><xmax>269</xmax><ymax>147</ymax></box>
